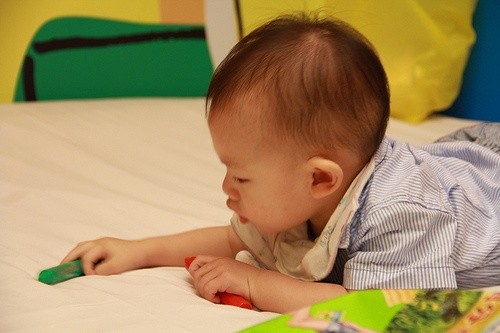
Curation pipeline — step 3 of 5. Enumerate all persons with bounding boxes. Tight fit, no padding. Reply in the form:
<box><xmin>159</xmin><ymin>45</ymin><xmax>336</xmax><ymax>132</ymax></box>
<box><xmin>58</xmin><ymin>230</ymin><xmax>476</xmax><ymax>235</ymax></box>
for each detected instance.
<box><xmin>57</xmin><ymin>6</ymin><xmax>499</xmax><ymax>321</ymax></box>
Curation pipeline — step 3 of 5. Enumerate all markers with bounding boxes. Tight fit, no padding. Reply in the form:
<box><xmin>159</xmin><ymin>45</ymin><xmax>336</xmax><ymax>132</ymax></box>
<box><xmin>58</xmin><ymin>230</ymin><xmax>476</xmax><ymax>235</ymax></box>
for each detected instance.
<box><xmin>38</xmin><ymin>259</ymin><xmax>88</xmax><ymax>286</ymax></box>
<box><xmin>184</xmin><ymin>256</ymin><xmax>253</xmax><ymax>311</ymax></box>
